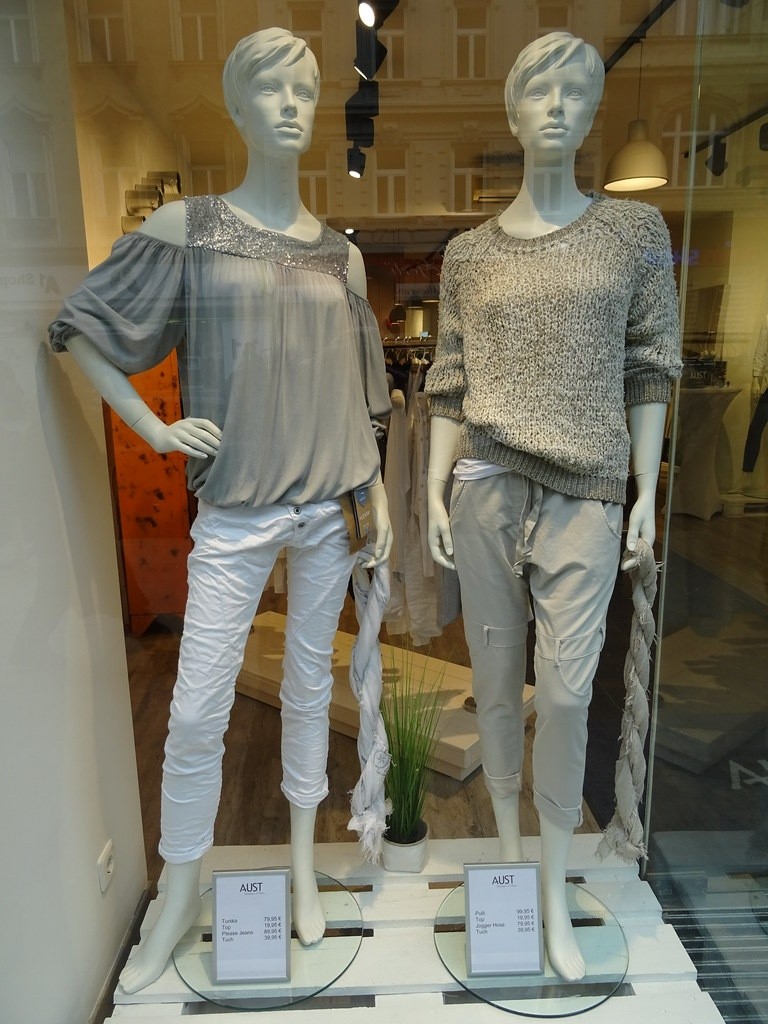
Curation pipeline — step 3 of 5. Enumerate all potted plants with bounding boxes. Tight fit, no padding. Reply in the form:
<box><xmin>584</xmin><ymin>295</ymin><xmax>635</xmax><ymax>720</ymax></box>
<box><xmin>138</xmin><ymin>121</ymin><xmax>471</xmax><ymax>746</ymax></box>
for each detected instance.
<box><xmin>374</xmin><ymin>632</ymin><xmax>455</xmax><ymax>871</ymax></box>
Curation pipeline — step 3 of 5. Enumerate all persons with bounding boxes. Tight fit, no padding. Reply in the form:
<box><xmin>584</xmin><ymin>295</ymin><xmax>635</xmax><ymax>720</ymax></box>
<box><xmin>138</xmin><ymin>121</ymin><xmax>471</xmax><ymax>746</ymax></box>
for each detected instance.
<box><xmin>48</xmin><ymin>26</ymin><xmax>392</xmax><ymax>993</ymax></box>
<box><xmin>728</xmin><ymin>317</ymin><xmax>768</xmax><ymax>494</ymax></box>
<box><xmin>424</xmin><ymin>30</ymin><xmax>683</xmax><ymax>979</ymax></box>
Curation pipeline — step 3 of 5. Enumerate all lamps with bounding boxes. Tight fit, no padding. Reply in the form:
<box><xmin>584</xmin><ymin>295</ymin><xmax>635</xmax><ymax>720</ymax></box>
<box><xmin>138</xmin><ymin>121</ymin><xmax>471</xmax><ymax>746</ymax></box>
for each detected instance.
<box><xmin>388</xmin><ymin>310</ymin><xmax>400</xmax><ymax>325</ymax></box>
<box><xmin>346</xmin><ymin>148</ymin><xmax>366</xmax><ymax>178</ymax></box>
<box><xmin>354</xmin><ymin>39</ymin><xmax>388</xmax><ymax>81</ymax></box>
<box><xmin>421</xmin><ymin>284</ymin><xmax>439</xmax><ymax>303</ymax></box>
<box><xmin>406</xmin><ymin>290</ymin><xmax>423</xmax><ymax>309</ymax></box>
<box><xmin>356</xmin><ymin>0</ymin><xmax>399</xmax><ymax>30</ymax></box>
<box><xmin>389</xmin><ymin>304</ymin><xmax>406</xmax><ymax>322</ymax></box>
<box><xmin>602</xmin><ymin>119</ymin><xmax>669</xmax><ymax>192</ymax></box>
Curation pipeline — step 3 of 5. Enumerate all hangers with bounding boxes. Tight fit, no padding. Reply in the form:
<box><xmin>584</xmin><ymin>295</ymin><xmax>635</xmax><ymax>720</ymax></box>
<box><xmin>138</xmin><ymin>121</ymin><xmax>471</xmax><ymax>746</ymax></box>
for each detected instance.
<box><xmin>383</xmin><ymin>335</ymin><xmax>434</xmax><ymax>365</ymax></box>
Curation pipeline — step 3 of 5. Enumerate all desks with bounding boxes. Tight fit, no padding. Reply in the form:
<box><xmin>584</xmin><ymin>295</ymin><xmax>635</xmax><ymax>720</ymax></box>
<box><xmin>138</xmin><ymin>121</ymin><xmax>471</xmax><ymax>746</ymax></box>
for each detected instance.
<box><xmin>660</xmin><ymin>384</ymin><xmax>743</xmax><ymax>522</ymax></box>
